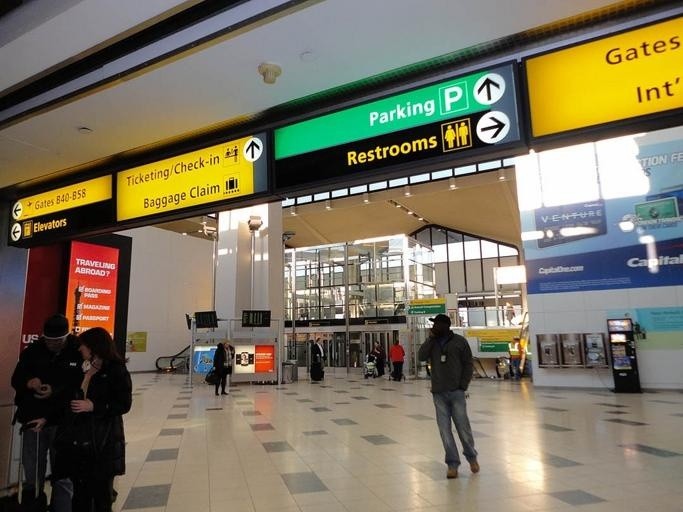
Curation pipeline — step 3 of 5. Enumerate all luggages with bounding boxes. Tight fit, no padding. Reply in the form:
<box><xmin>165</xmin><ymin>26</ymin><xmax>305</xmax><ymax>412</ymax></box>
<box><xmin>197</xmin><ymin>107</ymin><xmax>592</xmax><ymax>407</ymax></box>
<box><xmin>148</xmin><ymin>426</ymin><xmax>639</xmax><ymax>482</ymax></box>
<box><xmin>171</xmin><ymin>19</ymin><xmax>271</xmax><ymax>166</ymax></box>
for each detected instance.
<box><xmin>311</xmin><ymin>362</ymin><xmax>322</xmax><ymax>381</ymax></box>
<box><xmin>1</xmin><ymin>482</ymin><xmax>47</xmax><ymax>511</ymax></box>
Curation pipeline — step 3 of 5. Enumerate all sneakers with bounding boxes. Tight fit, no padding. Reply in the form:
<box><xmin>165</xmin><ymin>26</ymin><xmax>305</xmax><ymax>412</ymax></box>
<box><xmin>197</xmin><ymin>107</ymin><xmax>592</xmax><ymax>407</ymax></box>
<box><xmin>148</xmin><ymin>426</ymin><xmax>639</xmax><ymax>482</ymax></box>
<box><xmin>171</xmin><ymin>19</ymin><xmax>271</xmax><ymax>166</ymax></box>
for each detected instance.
<box><xmin>215</xmin><ymin>391</ymin><xmax>228</xmax><ymax>395</ymax></box>
<box><xmin>470</xmin><ymin>459</ymin><xmax>479</xmax><ymax>473</ymax></box>
<box><xmin>447</xmin><ymin>464</ymin><xmax>457</xmax><ymax>479</ymax></box>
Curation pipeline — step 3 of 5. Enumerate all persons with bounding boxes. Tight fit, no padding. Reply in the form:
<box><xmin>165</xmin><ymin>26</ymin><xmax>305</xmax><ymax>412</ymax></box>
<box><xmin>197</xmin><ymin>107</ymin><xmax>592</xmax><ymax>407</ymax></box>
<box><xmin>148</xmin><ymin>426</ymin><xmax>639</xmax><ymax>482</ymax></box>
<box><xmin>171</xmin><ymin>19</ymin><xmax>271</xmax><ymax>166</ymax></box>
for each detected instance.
<box><xmin>312</xmin><ymin>336</ymin><xmax>326</xmax><ymax>368</ymax></box>
<box><xmin>504</xmin><ymin>301</ymin><xmax>515</xmax><ymax>325</ymax></box>
<box><xmin>388</xmin><ymin>338</ymin><xmax>404</xmax><ymax>381</ymax></box>
<box><xmin>24</xmin><ymin>324</ymin><xmax>131</xmax><ymax>511</ymax></box>
<box><xmin>211</xmin><ymin>339</ymin><xmax>235</xmax><ymax>395</ymax></box>
<box><xmin>371</xmin><ymin>339</ymin><xmax>385</xmax><ymax>377</ymax></box>
<box><xmin>417</xmin><ymin>313</ymin><xmax>480</xmax><ymax>480</ymax></box>
<box><xmin>505</xmin><ymin>335</ymin><xmax>526</xmax><ymax>379</ymax></box>
<box><xmin>8</xmin><ymin>316</ymin><xmax>81</xmax><ymax>510</ymax></box>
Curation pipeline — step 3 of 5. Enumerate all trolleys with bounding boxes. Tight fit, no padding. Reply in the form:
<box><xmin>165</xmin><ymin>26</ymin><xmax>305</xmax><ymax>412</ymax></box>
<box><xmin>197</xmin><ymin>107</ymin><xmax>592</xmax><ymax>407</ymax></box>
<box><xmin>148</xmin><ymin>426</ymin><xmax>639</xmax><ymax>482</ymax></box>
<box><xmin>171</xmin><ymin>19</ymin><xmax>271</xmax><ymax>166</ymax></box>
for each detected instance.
<box><xmin>363</xmin><ymin>354</ymin><xmax>378</xmax><ymax>379</ymax></box>
<box><xmin>389</xmin><ymin>370</ymin><xmax>405</xmax><ymax>381</ymax></box>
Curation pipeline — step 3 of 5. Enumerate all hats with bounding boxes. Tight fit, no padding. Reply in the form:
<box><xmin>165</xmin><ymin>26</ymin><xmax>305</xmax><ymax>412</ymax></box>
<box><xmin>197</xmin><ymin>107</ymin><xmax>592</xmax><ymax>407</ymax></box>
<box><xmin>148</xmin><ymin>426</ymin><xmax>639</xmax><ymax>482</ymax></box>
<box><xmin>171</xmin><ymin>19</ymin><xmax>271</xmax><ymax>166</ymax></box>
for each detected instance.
<box><xmin>43</xmin><ymin>314</ymin><xmax>69</xmax><ymax>341</ymax></box>
<box><xmin>429</xmin><ymin>314</ymin><xmax>451</xmax><ymax>327</ymax></box>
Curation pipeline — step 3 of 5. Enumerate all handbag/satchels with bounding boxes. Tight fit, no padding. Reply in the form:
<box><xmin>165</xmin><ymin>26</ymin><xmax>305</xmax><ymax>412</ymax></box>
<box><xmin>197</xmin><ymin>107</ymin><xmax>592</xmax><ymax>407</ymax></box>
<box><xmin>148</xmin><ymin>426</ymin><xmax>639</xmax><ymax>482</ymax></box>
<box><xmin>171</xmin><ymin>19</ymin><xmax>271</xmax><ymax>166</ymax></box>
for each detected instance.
<box><xmin>205</xmin><ymin>368</ymin><xmax>215</xmax><ymax>384</ymax></box>
